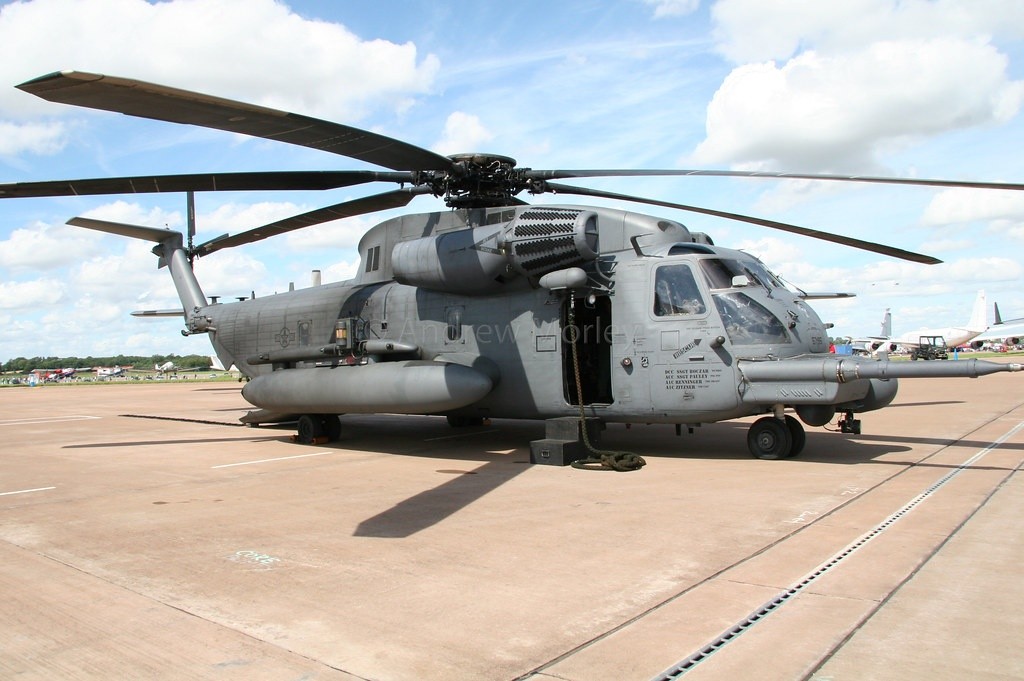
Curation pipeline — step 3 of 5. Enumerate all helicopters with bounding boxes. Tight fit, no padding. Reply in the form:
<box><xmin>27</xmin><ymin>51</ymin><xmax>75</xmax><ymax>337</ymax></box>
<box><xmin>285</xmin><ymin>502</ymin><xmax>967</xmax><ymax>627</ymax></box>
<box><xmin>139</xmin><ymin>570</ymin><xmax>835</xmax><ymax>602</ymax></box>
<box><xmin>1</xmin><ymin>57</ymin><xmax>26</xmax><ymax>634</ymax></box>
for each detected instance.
<box><xmin>0</xmin><ymin>70</ymin><xmax>1024</xmax><ymax>461</ymax></box>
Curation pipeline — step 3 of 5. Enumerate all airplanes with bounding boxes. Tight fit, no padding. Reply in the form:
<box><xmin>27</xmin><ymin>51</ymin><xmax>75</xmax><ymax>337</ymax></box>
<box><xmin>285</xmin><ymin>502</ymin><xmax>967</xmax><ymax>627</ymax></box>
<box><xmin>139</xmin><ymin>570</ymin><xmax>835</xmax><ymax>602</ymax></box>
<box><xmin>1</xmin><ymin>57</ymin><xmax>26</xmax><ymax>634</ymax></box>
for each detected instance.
<box><xmin>154</xmin><ymin>361</ymin><xmax>179</xmax><ymax>376</ymax></box>
<box><xmin>851</xmin><ymin>287</ymin><xmax>1024</xmax><ymax>361</ymax></box>
